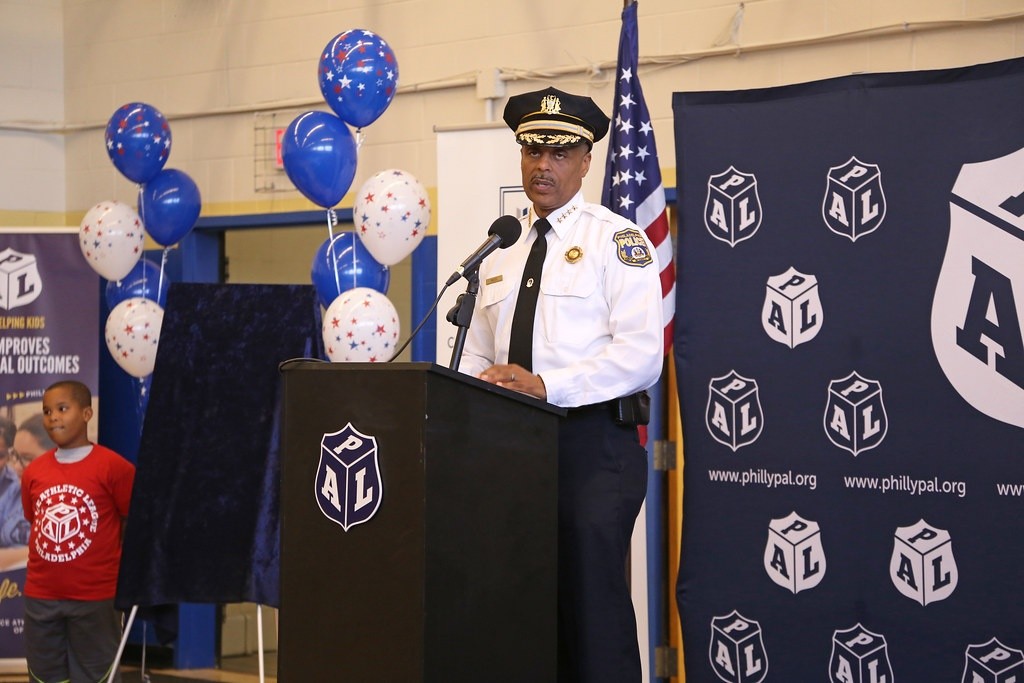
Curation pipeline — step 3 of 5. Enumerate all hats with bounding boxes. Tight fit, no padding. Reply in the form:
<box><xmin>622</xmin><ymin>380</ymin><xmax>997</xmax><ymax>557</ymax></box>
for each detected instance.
<box><xmin>503</xmin><ymin>86</ymin><xmax>610</xmax><ymax>153</ymax></box>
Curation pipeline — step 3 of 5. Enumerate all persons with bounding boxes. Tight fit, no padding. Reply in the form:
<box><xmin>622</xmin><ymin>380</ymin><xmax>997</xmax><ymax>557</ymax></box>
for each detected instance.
<box><xmin>0</xmin><ymin>412</ymin><xmax>73</xmax><ymax>572</ymax></box>
<box><xmin>457</xmin><ymin>87</ymin><xmax>666</xmax><ymax>683</ymax></box>
<box><xmin>19</xmin><ymin>378</ymin><xmax>138</xmax><ymax>683</ymax></box>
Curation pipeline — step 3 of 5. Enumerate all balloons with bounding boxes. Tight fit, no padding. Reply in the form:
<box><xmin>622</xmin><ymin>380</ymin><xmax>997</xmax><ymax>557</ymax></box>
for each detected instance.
<box><xmin>280</xmin><ymin>26</ymin><xmax>430</xmax><ymax>362</ymax></box>
<box><xmin>76</xmin><ymin>102</ymin><xmax>202</xmax><ymax>379</ymax></box>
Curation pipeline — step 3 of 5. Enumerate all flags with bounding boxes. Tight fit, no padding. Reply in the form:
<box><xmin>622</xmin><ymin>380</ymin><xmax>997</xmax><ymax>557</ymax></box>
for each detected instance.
<box><xmin>598</xmin><ymin>1</ymin><xmax>678</xmax><ymax>449</ymax></box>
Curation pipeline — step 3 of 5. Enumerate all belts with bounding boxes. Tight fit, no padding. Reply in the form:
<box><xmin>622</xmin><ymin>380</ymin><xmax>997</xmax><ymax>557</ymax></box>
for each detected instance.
<box><xmin>588</xmin><ymin>403</ymin><xmax>613</xmax><ymax>411</ymax></box>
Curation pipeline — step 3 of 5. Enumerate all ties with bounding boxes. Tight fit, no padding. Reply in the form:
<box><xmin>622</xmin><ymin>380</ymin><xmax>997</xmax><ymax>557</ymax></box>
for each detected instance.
<box><xmin>507</xmin><ymin>217</ymin><xmax>552</xmax><ymax>374</ymax></box>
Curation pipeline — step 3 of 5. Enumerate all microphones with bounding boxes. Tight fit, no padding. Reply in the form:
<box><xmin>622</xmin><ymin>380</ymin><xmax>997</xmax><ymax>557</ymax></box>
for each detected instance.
<box><xmin>445</xmin><ymin>215</ymin><xmax>522</xmax><ymax>285</ymax></box>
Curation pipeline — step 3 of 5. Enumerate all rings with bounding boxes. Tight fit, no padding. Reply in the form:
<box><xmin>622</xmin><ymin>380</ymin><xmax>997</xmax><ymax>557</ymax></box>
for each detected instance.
<box><xmin>511</xmin><ymin>373</ymin><xmax>514</xmax><ymax>381</ymax></box>
